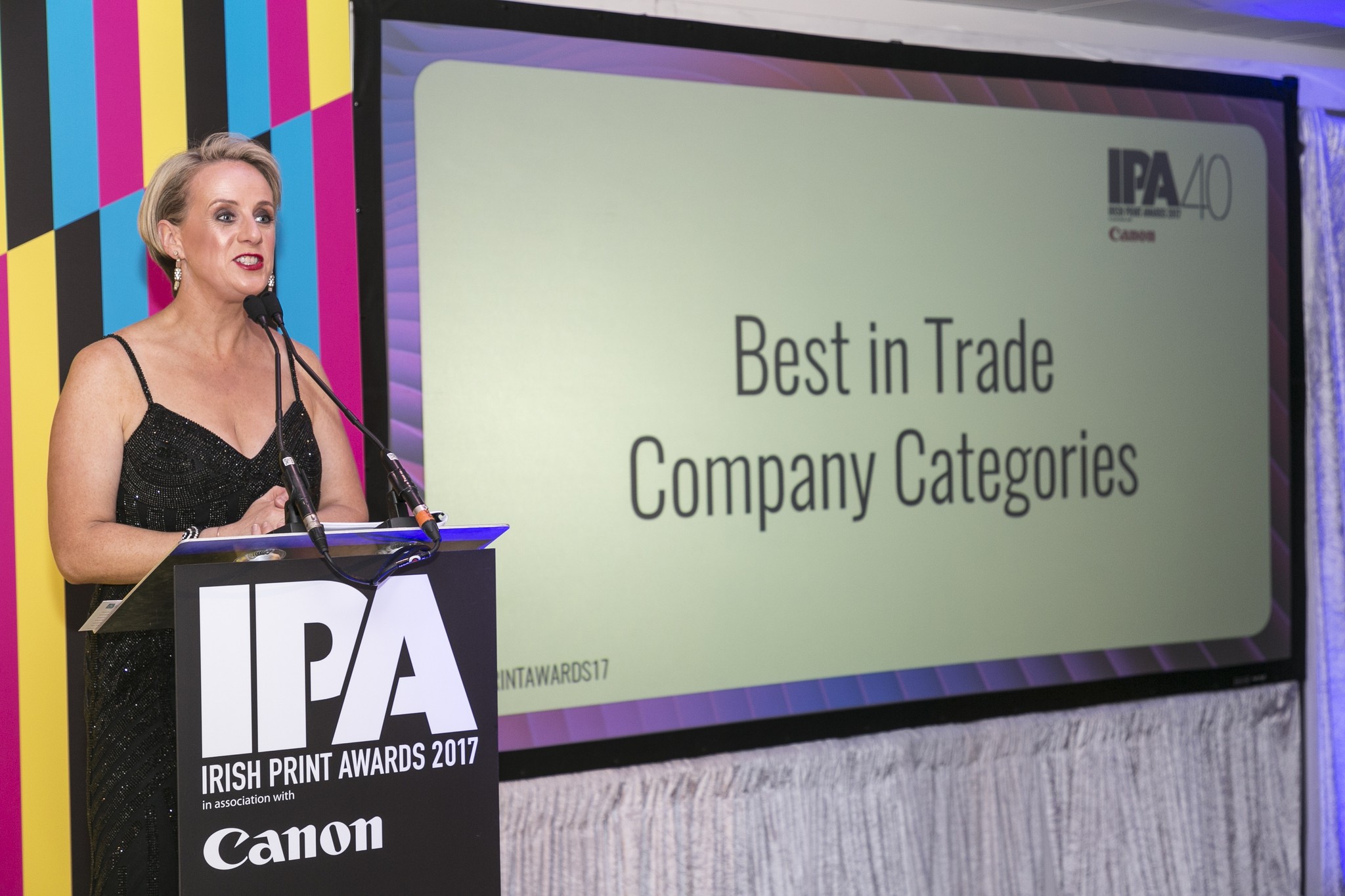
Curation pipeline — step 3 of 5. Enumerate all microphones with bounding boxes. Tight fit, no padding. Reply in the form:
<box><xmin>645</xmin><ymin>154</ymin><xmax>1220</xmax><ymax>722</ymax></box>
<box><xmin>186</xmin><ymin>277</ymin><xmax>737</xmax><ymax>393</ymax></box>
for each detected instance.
<box><xmin>243</xmin><ymin>294</ymin><xmax>327</xmax><ymax>554</ymax></box>
<box><xmin>261</xmin><ymin>290</ymin><xmax>440</xmax><ymax>541</ymax></box>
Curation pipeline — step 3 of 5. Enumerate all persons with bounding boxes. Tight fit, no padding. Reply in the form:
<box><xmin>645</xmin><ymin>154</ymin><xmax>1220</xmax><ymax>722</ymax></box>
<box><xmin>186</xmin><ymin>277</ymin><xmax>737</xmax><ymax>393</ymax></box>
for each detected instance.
<box><xmin>48</xmin><ymin>136</ymin><xmax>367</xmax><ymax>896</ymax></box>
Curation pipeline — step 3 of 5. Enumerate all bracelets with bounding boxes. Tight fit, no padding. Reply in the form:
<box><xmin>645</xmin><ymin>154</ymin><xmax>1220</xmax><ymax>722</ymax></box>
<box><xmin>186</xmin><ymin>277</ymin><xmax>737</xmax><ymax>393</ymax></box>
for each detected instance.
<box><xmin>178</xmin><ymin>525</ymin><xmax>206</xmax><ymax>544</ymax></box>
<box><xmin>217</xmin><ymin>526</ymin><xmax>221</xmax><ymax>537</ymax></box>
